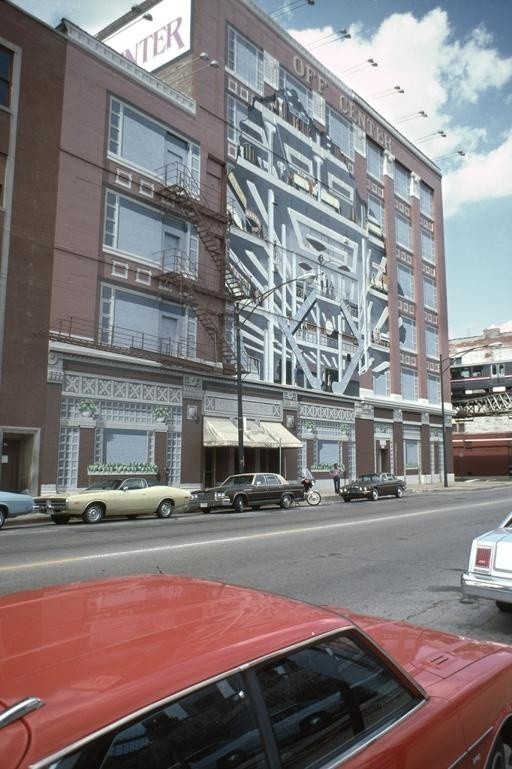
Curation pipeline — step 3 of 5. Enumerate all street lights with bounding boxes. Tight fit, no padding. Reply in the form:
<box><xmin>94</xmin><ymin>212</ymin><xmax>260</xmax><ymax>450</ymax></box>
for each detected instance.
<box><xmin>233</xmin><ymin>272</ymin><xmax>316</xmax><ymax>483</ymax></box>
<box><xmin>439</xmin><ymin>341</ymin><xmax>503</xmax><ymax>488</ymax></box>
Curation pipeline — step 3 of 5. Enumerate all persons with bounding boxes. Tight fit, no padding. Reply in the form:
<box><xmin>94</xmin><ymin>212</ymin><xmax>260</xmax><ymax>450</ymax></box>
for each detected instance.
<box><xmin>329</xmin><ymin>463</ymin><xmax>344</xmax><ymax>494</ymax></box>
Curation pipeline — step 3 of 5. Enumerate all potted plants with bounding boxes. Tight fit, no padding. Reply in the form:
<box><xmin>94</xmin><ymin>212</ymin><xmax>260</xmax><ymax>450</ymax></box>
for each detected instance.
<box><xmin>339</xmin><ymin>422</ymin><xmax>353</xmax><ymax>438</ymax></box>
<box><xmin>152</xmin><ymin>408</ymin><xmax>170</xmax><ymax>425</ymax></box>
<box><xmin>86</xmin><ymin>459</ymin><xmax>158</xmax><ymax>478</ymax></box>
<box><xmin>74</xmin><ymin>398</ymin><xmax>101</xmax><ymax>418</ymax></box>
<box><xmin>311</xmin><ymin>463</ymin><xmax>345</xmax><ymax>476</ymax></box>
<box><xmin>303</xmin><ymin>417</ymin><xmax>319</xmax><ymax>435</ymax></box>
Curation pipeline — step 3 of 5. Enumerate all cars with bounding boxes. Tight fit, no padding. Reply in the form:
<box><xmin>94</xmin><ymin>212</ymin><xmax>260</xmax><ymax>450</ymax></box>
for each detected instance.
<box><xmin>0</xmin><ymin>572</ymin><xmax>512</xmax><ymax>769</ymax></box>
<box><xmin>339</xmin><ymin>472</ymin><xmax>407</xmax><ymax>502</ymax></box>
<box><xmin>0</xmin><ymin>490</ymin><xmax>35</xmax><ymax>528</ymax></box>
<box><xmin>460</xmin><ymin>511</ymin><xmax>512</xmax><ymax>614</ymax></box>
<box><xmin>33</xmin><ymin>476</ymin><xmax>190</xmax><ymax>525</ymax></box>
<box><xmin>189</xmin><ymin>472</ymin><xmax>305</xmax><ymax>514</ymax></box>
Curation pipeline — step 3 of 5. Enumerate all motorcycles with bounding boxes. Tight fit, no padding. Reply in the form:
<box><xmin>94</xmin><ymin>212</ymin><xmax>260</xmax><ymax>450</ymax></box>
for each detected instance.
<box><xmin>294</xmin><ymin>467</ymin><xmax>322</xmax><ymax>506</ymax></box>
